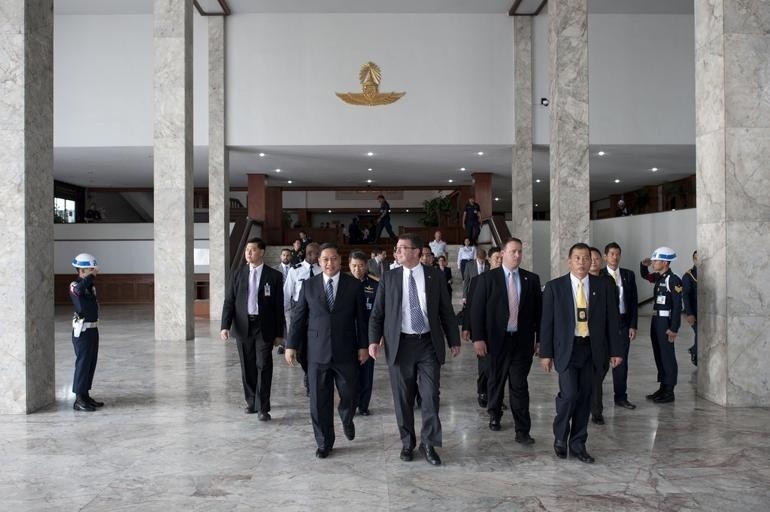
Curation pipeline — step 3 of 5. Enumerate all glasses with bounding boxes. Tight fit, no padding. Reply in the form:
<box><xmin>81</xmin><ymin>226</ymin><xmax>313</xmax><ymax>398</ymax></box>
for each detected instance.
<box><xmin>393</xmin><ymin>246</ymin><xmax>418</xmax><ymax>252</ymax></box>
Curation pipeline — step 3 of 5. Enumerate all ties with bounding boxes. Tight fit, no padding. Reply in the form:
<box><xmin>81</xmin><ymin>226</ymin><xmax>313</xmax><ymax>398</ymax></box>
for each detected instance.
<box><xmin>326</xmin><ymin>278</ymin><xmax>335</xmax><ymax>314</ymax></box>
<box><xmin>613</xmin><ymin>271</ymin><xmax>619</xmax><ymax>287</ymax></box>
<box><xmin>283</xmin><ymin>267</ymin><xmax>288</xmax><ymax>285</ymax></box>
<box><xmin>250</xmin><ymin>269</ymin><xmax>258</xmax><ymax>314</ymax></box>
<box><xmin>480</xmin><ymin>264</ymin><xmax>483</xmax><ymax>272</ymax></box>
<box><xmin>310</xmin><ymin>264</ymin><xmax>315</xmax><ymax>279</ymax></box>
<box><xmin>408</xmin><ymin>270</ymin><xmax>425</xmax><ymax>333</ymax></box>
<box><xmin>576</xmin><ymin>280</ymin><xmax>588</xmax><ymax>339</ymax></box>
<box><xmin>508</xmin><ymin>271</ymin><xmax>519</xmax><ymax>328</ymax></box>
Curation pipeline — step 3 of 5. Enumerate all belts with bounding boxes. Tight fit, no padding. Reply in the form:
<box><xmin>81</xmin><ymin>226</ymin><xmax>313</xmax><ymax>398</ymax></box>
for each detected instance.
<box><xmin>247</xmin><ymin>315</ymin><xmax>258</xmax><ymax>322</ymax></box>
<box><xmin>652</xmin><ymin>309</ymin><xmax>670</xmax><ymax>317</ymax></box>
<box><xmin>401</xmin><ymin>332</ymin><xmax>433</xmax><ymax>340</ymax></box>
<box><xmin>72</xmin><ymin>323</ymin><xmax>97</xmax><ymax>328</ymax></box>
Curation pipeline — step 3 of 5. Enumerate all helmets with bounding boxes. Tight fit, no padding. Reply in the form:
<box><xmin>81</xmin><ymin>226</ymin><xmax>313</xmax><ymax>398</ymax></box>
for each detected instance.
<box><xmin>650</xmin><ymin>247</ymin><xmax>678</xmax><ymax>261</ymax></box>
<box><xmin>71</xmin><ymin>253</ymin><xmax>98</xmax><ymax>269</ymax></box>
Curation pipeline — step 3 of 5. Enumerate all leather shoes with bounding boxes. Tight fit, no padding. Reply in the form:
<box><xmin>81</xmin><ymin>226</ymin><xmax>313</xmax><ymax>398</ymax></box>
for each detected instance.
<box><xmin>554</xmin><ymin>438</ymin><xmax>568</xmax><ymax>458</ymax></box>
<box><xmin>343</xmin><ymin>421</ymin><xmax>355</xmax><ymax>441</ymax></box>
<box><xmin>654</xmin><ymin>391</ymin><xmax>674</xmax><ymax>403</ymax></box>
<box><xmin>489</xmin><ymin>415</ymin><xmax>502</xmax><ymax>432</ymax></box>
<box><xmin>88</xmin><ymin>399</ymin><xmax>105</xmax><ymax>407</ymax></box>
<box><xmin>419</xmin><ymin>442</ymin><xmax>441</xmax><ymax>465</ymax></box>
<box><xmin>478</xmin><ymin>393</ymin><xmax>488</xmax><ymax>409</ymax></box>
<box><xmin>592</xmin><ymin>417</ymin><xmax>604</xmax><ymax>425</ymax></box>
<box><xmin>400</xmin><ymin>446</ymin><xmax>414</xmax><ymax>462</ymax></box>
<box><xmin>615</xmin><ymin>400</ymin><xmax>635</xmax><ymax>410</ymax></box>
<box><xmin>278</xmin><ymin>347</ymin><xmax>283</xmax><ymax>354</ymax></box>
<box><xmin>73</xmin><ymin>399</ymin><xmax>96</xmax><ymax>412</ymax></box>
<box><xmin>515</xmin><ymin>435</ymin><xmax>535</xmax><ymax>445</ymax></box>
<box><xmin>358</xmin><ymin>406</ymin><xmax>370</xmax><ymax>416</ymax></box>
<box><xmin>569</xmin><ymin>450</ymin><xmax>595</xmax><ymax>464</ymax></box>
<box><xmin>688</xmin><ymin>348</ymin><xmax>697</xmax><ymax>366</ymax></box>
<box><xmin>244</xmin><ymin>407</ymin><xmax>258</xmax><ymax>414</ymax></box>
<box><xmin>258</xmin><ymin>411</ymin><xmax>271</xmax><ymax>422</ymax></box>
<box><xmin>501</xmin><ymin>404</ymin><xmax>507</xmax><ymax>411</ymax></box>
<box><xmin>648</xmin><ymin>389</ymin><xmax>664</xmax><ymax>399</ymax></box>
<box><xmin>316</xmin><ymin>445</ymin><xmax>333</xmax><ymax>459</ymax></box>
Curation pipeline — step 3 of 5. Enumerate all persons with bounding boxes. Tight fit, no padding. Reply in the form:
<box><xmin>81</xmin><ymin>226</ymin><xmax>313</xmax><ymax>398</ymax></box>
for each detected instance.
<box><xmin>462</xmin><ymin>195</ymin><xmax>483</xmax><ymax>246</ymax></box>
<box><xmin>458</xmin><ymin>237</ymin><xmax>475</xmax><ymax>283</ymax></box>
<box><xmin>299</xmin><ymin>230</ymin><xmax>312</xmax><ymax>251</ymax></box>
<box><xmin>270</xmin><ymin>248</ymin><xmax>299</xmax><ymax>354</ymax></box>
<box><xmin>369</xmin><ymin>234</ymin><xmax>461</xmax><ymax>465</ymax></box>
<box><xmin>600</xmin><ymin>242</ymin><xmax>638</xmax><ymax>410</ymax></box>
<box><xmin>285</xmin><ymin>243</ymin><xmax>369</xmax><ymax>456</ymax></box>
<box><xmin>319</xmin><ymin>216</ymin><xmax>368</xmax><ymax>243</ymax></box>
<box><xmin>470</xmin><ymin>238</ymin><xmax>543</xmax><ymax>445</ymax></box>
<box><xmin>69</xmin><ymin>253</ymin><xmax>104</xmax><ymax>411</ymax></box>
<box><xmin>420</xmin><ymin>245</ymin><xmax>433</xmax><ymax>266</ymax></box>
<box><xmin>461</xmin><ymin>248</ymin><xmax>515</xmax><ymax>410</ymax></box>
<box><xmin>291</xmin><ymin>240</ymin><xmax>305</xmax><ymax>263</ymax></box>
<box><xmin>590</xmin><ymin>246</ymin><xmax>620</xmax><ymax>424</ymax></box>
<box><xmin>429</xmin><ymin>230</ymin><xmax>448</xmax><ymax>265</ymax></box>
<box><xmin>341</xmin><ymin>251</ymin><xmax>380</xmax><ymax>416</ymax></box>
<box><xmin>366</xmin><ymin>249</ymin><xmax>387</xmax><ymax>292</ymax></box>
<box><xmin>539</xmin><ymin>243</ymin><xmax>623</xmax><ymax>463</ymax></box>
<box><xmin>640</xmin><ymin>247</ymin><xmax>682</xmax><ymax>403</ymax></box>
<box><xmin>682</xmin><ymin>250</ymin><xmax>697</xmax><ymax>367</ymax></box>
<box><xmin>220</xmin><ymin>237</ymin><xmax>284</xmax><ymax>421</ymax></box>
<box><xmin>282</xmin><ymin>242</ymin><xmax>321</xmax><ymax>318</ymax></box>
<box><xmin>84</xmin><ymin>202</ymin><xmax>102</xmax><ymax>223</ymax></box>
<box><xmin>389</xmin><ymin>246</ymin><xmax>402</xmax><ymax>269</ymax></box>
<box><xmin>438</xmin><ymin>256</ymin><xmax>453</xmax><ymax>304</ymax></box>
<box><xmin>462</xmin><ymin>250</ymin><xmax>491</xmax><ymax>305</ymax></box>
<box><xmin>370</xmin><ymin>194</ymin><xmax>398</xmax><ymax>241</ymax></box>
